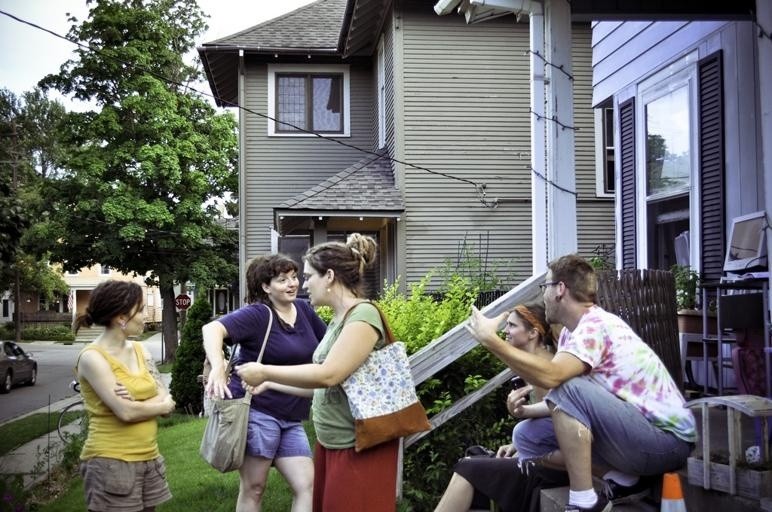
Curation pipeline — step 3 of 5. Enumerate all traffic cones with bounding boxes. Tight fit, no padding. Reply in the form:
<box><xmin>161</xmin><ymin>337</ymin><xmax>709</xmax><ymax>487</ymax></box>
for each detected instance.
<box><xmin>657</xmin><ymin>472</ymin><xmax>688</xmax><ymax>512</ymax></box>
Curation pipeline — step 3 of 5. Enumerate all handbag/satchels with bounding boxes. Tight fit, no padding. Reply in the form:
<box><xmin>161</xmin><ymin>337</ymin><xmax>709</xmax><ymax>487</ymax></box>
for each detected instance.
<box><xmin>335</xmin><ymin>300</ymin><xmax>433</xmax><ymax>454</ymax></box>
<box><xmin>197</xmin><ymin>386</ymin><xmax>256</xmax><ymax>474</ymax></box>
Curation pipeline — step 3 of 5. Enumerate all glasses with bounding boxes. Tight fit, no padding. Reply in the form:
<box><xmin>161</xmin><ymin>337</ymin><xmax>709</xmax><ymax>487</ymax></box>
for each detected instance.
<box><xmin>539</xmin><ymin>279</ymin><xmax>560</xmax><ymax>294</ymax></box>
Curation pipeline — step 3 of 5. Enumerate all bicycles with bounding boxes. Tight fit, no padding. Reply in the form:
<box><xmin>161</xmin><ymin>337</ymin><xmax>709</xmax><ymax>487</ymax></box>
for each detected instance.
<box><xmin>58</xmin><ymin>380</ymin><xmax>87</xmax><ymax>447</ymax></box>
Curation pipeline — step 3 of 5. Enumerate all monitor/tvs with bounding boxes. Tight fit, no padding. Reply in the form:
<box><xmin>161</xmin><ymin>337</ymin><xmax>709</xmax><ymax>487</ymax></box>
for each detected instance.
<box><xmin>723</xmin><ymin>210</ymin><xmax>766</xmax><ymax>271</ymax></box>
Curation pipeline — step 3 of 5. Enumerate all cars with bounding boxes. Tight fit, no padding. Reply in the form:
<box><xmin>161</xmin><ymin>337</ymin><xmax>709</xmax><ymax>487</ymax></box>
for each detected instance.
<box><xmin>0</xmin><ymin>341</ymin><xmax>38</xmax><ymax>395</ymax></box>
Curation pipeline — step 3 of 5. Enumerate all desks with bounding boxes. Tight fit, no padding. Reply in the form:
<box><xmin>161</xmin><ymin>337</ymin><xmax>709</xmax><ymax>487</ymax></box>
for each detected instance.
<box><xmin>695</xmin><ymin>276</ymin><xmax>772</xmax><ymax>399</ymax></box>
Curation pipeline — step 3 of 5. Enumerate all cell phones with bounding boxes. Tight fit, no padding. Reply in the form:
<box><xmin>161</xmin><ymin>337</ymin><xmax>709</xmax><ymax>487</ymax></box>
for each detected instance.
<box><xmin>510</xmin><ymin>376</ymin><xmax>530</xmax><ymax>402</ymax></box>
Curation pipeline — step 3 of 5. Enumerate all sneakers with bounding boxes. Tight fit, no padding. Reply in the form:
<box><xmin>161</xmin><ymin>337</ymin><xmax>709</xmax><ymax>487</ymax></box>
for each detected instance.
<box><xmin>562</xmin><ymin>494</ymin><xmax>613</xmax><ymax>512</ymax></box>
<box><xmin>597</xmin><ymin>475</ymin><xmax>651</xmax><ymax>505</ymax></box>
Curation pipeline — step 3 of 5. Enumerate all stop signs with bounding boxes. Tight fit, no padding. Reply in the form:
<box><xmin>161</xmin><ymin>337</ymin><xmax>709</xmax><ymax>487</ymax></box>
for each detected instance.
<box><xmin>175</xmin><ymin>294</ymin><xmax>191</xmax><ymax>310</ymax></box>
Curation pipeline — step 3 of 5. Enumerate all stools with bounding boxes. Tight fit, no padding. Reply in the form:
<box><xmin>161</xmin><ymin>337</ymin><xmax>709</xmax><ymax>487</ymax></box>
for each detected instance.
<box><xmin>678</xmin><ymin>332</ymin><xmax>723</xmax><ymax>399</ymax></box>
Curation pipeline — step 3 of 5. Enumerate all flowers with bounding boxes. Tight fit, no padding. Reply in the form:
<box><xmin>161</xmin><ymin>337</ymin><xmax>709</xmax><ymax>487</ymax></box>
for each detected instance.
<box><xmin>669</xmin><ymin>264</ymin><xmax>698</xmax><ymax>310</ymax></box>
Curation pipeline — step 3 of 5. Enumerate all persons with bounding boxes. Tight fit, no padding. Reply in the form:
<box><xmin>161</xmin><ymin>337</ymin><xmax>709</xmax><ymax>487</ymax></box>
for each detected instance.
<box><xmin>461</xmin><ymin>253</ymin><xmax>702</xmax><ymax>512</ymax></box>
<box><xmin>73</xmin><ymin>280</ymin><xmax>179</xmax><ymax>512</ymax></box>
<box><xmin>231</xmin><ymin>229</ymin><xmax>403</xmax><ymax>512</ymax></box>
<box><xmin>200</xmin><ymin>251</ymin><xmax>327</xmax><ymax>512</ymax></box>
<box><xmin>432</xmin><ymin>300</ymin><xmax>565</xmax><ymax>510</ymax></box>
